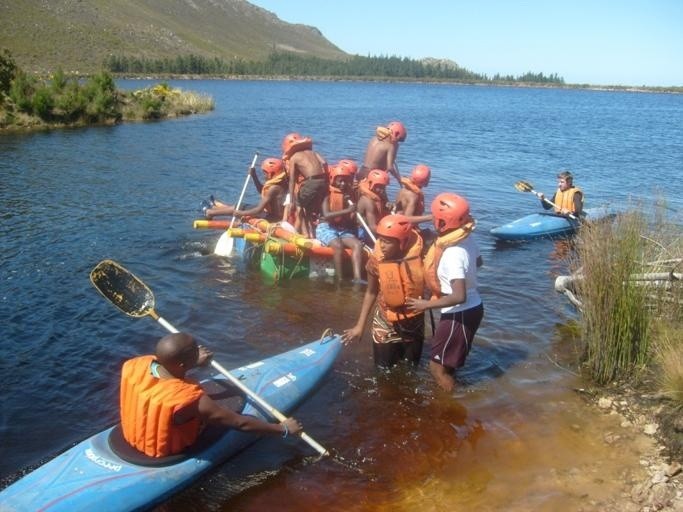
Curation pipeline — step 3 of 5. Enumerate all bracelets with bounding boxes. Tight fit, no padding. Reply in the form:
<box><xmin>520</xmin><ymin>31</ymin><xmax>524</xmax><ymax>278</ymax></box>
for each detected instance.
<box><xmin>281</xmin><ymin>422</ymin><xmax>289</xmax><ymax>439</ymax></box>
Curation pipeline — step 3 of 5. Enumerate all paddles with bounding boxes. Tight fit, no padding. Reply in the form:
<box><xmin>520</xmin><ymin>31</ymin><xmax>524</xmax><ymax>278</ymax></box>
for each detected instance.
<box><xmin>88</xmin><ymin>258</ymin><xmax>332</xmax><ymax>459</ymax></box>
<box><xmin>213</xmin><ymin>150</ymin><xmax>261</xmax><ymax>257</ymax></box>
<box><xmin>514</xmin><ymin>180</ymin><xmax>581</xmax><ymax>225</ymax></box>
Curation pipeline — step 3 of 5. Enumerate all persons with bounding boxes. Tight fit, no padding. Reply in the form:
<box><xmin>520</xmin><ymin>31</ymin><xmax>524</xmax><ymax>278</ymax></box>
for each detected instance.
<box><xmin>339</xmin><ymin>214</ymin><xmax>425</xmax><ymax>372</ymax></box>
<box><xmin>316</xmin><ymin>167</ymin><xmax>369</xmax><ymax>287</ymax></box>
<box><xmin>118</xmin><ymin>332</ymin><xmax>305</xmax><ymax>458</ymax></box>
<box><xmin>402</xmin><ymin>192</ymin><xmax>483</xmax><ymax>391</ymax></box>
<box><xmin>337</xmin><ymin>158</ymin><xmax>362</xmax><ymax>192</ymax></box>
<box><xmin>393</xmin><ymin>164</ymin><xmax>434</xmax><ymax>232</ymax></box>
<box><xmin>536</xmin><ymin>171</ymin><xmax>584</xmax><ymax>219</ymax></box>
<box><xmin>355</xmin><ymin>167</ymin><xmax>390</xmax><ymax>243</ymax></box>
<box><xmin>199</xmin><ymin>132</ymin><xmax>329</xmax><ymax>239</ymax></box>
<box><xmin>356</xmin><ymin>119</ymin><xmax>406</xmax><ymax>189</ymax></box>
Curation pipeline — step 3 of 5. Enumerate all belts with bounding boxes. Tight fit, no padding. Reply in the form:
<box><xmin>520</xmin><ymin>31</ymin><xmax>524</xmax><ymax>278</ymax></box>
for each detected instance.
<box><xmin>302</xmin><ymin>175</ymin><xmax>326</xmax><ymax>181</ymax></box>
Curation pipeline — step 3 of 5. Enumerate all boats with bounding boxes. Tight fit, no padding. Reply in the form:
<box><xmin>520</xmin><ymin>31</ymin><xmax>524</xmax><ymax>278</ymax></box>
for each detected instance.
<box><xmin>488</xmin><ymin>204</ymin><xmax>617</xmax><ymax>242</ymax></box>
<box><xmin>0</xmin><ymin>333</ymin><xmax>342</xmax><ymax>512</ymax></box>
<box><xmin>193</xmin><ymin>201</ymin><xmax>436</xmax><ymax>283</ymax></box>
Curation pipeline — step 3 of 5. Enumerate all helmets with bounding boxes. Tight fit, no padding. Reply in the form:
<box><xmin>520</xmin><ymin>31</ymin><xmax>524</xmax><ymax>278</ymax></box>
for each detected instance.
<box><xmin>375</xmin><ymin>214</ymin><xmax>412</xmax><ymax>251</ymax></box>
<box><xmin>330</xmin><ymin>166</ymin><xmax>349</xmax><ymax>186</ymax></box>
<box><xmin>281</xmin><ymin>133</ymin><xmax>302</xmax><ymax>153</ymax></box>
<box><xmin>386</xmin><ymin>121</ymin><xmax>406</xmax><ymax>143</ymax></box>
<box><xmin>410</xmin><ymin>165</ymin><xmax>431</xmax><ymax>187</ymax></box>
<box><xmin>431</xmin><ymin>193</ymin><xmax>471</xmax><ymax>232</ymax></box>
<box><xmin>261</xmin><ymin>158</ymin><xmax>282</xmax><ymax>179</ymax></box>
<box><xmin>339</xmin><ymin>160</ymin><xmax>357</xmax><ymax>174</ymax></box>
<box><xmin>367</xmin><ymin>168</ymin><xmax>389</xmax><ymax>191</ymax></box>
